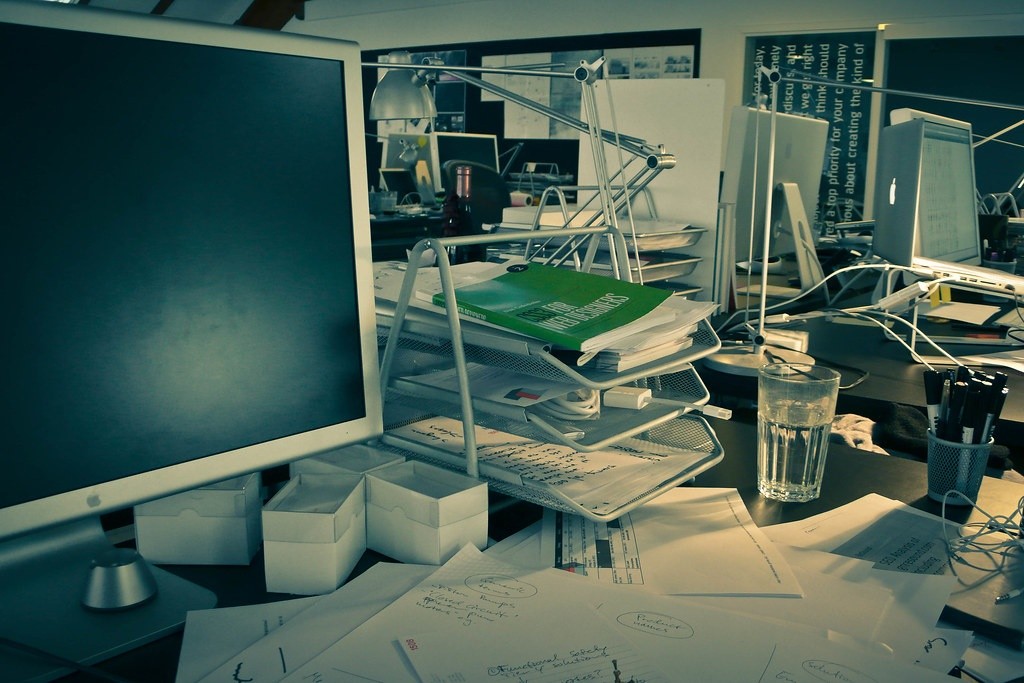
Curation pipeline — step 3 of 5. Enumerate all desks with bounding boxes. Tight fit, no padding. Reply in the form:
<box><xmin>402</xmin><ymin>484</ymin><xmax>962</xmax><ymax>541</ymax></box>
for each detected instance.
<box><xmin>0</xmin><ymin>417</ymin><xmax>1024</xmax><ymax>683</ymax></box>
<box><xmin>703</xmin><ymin>293</ymin><xmax>1024</xmax><ymax>426</ymax></box>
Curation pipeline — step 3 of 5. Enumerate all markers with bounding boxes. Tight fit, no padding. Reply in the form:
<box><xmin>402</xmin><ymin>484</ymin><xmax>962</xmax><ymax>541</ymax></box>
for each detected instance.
<box><xmin>922</xmin><ymin>366</ymin><xmax>1009</xmax><ymax>504</ymax></box>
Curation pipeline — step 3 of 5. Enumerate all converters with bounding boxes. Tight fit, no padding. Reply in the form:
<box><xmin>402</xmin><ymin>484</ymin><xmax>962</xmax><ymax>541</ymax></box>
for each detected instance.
<box><xmin>879</xmin><ymin>283</ymin><xmax>929</xmax><ymax>311</ymax></box>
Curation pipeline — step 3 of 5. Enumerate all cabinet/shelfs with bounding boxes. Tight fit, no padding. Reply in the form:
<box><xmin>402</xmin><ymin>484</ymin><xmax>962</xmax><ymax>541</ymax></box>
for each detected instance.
<box><xmin>376</xmin><ymin>226</ymin><xmax>725</xmax><ymax>523</ymax></box>
<box><xmin>487</xmin><ymin>183</ymin><xmax>707</xmax><ymax>303</ymax></box>
<box><xmin>508</xmin><ymin>163</ymin><xmax>575</xmax><ymax>199</ymax></box>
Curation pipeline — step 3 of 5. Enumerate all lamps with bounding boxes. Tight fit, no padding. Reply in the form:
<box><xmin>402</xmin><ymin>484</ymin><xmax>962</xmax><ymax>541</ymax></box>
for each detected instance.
<box><xmin>368</xmin><ymin>56</ymin><xmax>677</xmax><ymax>269</ymax></box>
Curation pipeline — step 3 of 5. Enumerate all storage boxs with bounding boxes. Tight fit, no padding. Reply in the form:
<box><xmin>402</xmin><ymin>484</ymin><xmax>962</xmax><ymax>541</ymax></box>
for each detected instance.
<box><xmin>133</xmin><ymin>441</ymin><xmax>490</xmax><ymax>596</ymax></box>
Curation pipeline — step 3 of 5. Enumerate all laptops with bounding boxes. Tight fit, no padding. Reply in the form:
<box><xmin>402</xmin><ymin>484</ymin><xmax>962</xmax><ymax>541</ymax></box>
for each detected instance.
<box><xmin>872</xmin><ymin>117</ymin><xmax>1024</xmax><ymax>296</ymax></box>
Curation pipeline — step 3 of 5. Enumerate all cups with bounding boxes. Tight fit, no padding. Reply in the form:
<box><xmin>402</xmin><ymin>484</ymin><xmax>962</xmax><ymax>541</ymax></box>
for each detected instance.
<box><xmin>756</xmin><ymin>362</ymin><xmax>841</xmax><ymax>502</ymax></box>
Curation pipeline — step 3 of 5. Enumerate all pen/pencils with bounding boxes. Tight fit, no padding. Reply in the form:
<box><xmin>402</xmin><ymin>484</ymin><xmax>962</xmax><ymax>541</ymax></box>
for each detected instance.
<box><xmin>994</xmin><ymin>585</ymin><xmax>1024</xmax><ymax>604</ymax></box>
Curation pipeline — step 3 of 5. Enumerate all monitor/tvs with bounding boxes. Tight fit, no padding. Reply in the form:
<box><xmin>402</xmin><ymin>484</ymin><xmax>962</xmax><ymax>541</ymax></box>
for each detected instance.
<box><xmin>890</xmin><ymin>108</ymin><xmax>981</xmax><ymax>286</ymax></box>
<box><xmin>379</xmin><ymin>130</ymin><xmax>500</xmax><ymax>194</ymax></box>
<box><xmin>0</xmin><ymin>0</ymin><xmax>383</xmax><ymax>666</ymax></box>
<box><xmin>722</xmin><ymin>106</ymin><xmax>829</xmax><ymax>299</ymax></box>
